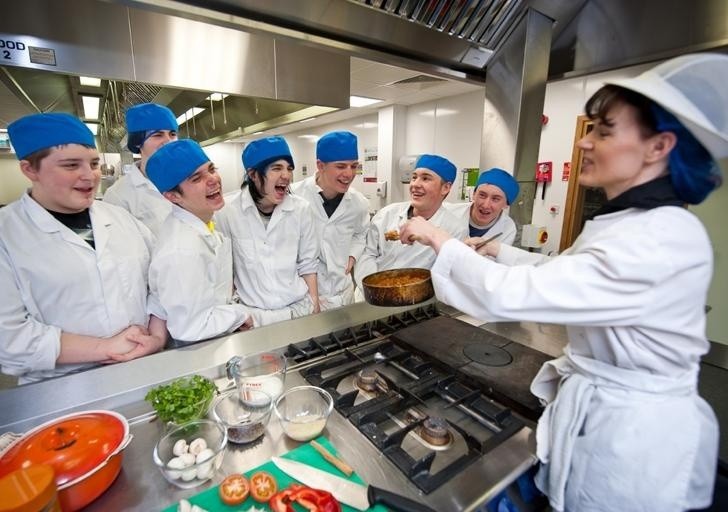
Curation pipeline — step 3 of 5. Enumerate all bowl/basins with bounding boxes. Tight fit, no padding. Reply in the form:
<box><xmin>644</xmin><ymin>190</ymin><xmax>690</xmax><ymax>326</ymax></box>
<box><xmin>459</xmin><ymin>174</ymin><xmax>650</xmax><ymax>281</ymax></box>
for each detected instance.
<box><xmin>274</xmin><ymin>384</ymin><xmax>333</xmax><ymax>441</ymax></box>
<box><xmin>152</xmin><ymin>419</ymin><xmax>226</xmax><ymax>490</ymax></box>
<box><xmin>213</xmin><ymin>388</ymin><xmax>273</xmax><ymax>444</ymax></box>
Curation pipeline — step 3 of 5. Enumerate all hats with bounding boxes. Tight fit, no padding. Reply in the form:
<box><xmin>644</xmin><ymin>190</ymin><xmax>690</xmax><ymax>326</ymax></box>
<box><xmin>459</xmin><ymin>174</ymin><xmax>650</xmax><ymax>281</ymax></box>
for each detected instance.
<box><xmin>601</xmin><ymin>50</ymin><xmax>728</xmax><ymax>162</ymax></box>
<box><xmin>124</xmin><ymin>102</ymin><xmax>179</xmax><ymax>133</ymax></box>
<box><xmin>6</xmin><ymin>112</ymin><xmax>97</xmax><ymax>161</ymax></box>
<box><xmin>145</xmin><ymin>139</ymin><xmax>211</xmax><ymax>194</ymax></box>
<box><xmin>474</xmin><ymin>167</ymin><xmax>520</xmax><ymax>206</ymax></box>
<box><xmin>316</xmin><ymin>131</ymin><xmax>359</xmax><ymax>163</ymax></box>
<box><xmin>415</xmin><ymin>154</ymin><xmax>457</xmax><ymax>185</ymax></box>
<box><xmin>241</xmin><ymin>135</ymin><xmax>292</xmax><ymax>171</ymax></box>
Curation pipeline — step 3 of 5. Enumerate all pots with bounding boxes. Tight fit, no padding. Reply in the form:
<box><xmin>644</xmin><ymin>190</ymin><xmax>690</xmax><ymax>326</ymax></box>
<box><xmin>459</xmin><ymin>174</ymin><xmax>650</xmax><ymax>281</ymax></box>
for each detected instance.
<box><xmin>360</xmin><ymin>231</ymin><xmax>505</xmax><ymax>305</ymax></box>
<box><xmin>1</xmin><ymin>405</ymin><xmax>131</xmax><ymax>511</ymax></box>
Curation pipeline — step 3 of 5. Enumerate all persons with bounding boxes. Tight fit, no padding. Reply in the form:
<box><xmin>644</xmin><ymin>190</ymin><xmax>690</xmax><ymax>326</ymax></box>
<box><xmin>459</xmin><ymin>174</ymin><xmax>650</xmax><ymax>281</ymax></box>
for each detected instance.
<box><xmin>99</xmin><ymin>101</ymin><xmax>180</xmax><ymax>236</ymax></box>
<box><xmin>286</xmin><ymin>131</ymin><xmax>374</xmax><ymax>320</ymax></box>
<box><xmin>212</xmin><ymin>135</ymin><xmax>322</xmax><ymax>334</ymax></box>
<box><xmin>397</xmin><ymin>51</ymin><xmax>728</xmax><ymax>512</ymax></box>
<box><xmin>442</xmin><ymin>167</ymin><xmax>524</xmax><ymax>264</ymax></box>
<box><xmin>350</xmin><ymin>153</ymin><xmax>464</xmax><ymax>307</ymax></box>
<box><xmin>0</xmin><ymin>110</ymin><xmax>169</xmax><ymax>390</ymax></box>
<box><xmin>143</xmin><ymin>138</ymin><xmax>256</xmax><ymax>346</ymax></box>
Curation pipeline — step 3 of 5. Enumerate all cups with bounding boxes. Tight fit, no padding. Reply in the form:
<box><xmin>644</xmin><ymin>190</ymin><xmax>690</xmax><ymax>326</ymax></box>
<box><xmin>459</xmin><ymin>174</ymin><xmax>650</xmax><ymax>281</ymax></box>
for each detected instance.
<box><xmin>224</xmin><ymin>351</ymin><xmax>287</xmax><ymax>407</ymax></box>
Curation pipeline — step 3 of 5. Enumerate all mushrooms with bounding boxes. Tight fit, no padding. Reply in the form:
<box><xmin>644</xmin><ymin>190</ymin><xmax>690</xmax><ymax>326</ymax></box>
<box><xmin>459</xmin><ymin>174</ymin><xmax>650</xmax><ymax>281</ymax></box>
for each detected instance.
<box><xmin>164</xmin><ymin>438</ymin><xmax>216</xmax><ymax>483</ymax></box>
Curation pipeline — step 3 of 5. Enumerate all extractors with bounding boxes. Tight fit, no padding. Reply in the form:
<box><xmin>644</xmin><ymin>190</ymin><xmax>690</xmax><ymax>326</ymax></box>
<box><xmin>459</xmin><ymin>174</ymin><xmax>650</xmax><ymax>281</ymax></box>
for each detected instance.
<box><xmin>1</xmin><ymin>2</ymin><xmax>536</xmax><ymax>158</ymax></box>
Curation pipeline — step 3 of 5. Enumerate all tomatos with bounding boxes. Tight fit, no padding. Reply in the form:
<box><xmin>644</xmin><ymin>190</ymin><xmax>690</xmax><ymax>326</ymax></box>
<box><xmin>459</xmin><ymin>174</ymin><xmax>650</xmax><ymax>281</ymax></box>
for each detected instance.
<box><xmin>220</xmin><ymin>472</ymin><xmax>278</xmax><ymax>505</ymax></box>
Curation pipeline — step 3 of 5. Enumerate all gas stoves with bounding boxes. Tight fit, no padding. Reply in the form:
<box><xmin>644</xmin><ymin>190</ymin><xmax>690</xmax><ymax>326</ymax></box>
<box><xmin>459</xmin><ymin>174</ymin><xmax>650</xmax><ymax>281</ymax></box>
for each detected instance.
<box><xmin>293</xmin><ymin>310</ymin><xmax>569</xmax><ymax>498</ymax></box>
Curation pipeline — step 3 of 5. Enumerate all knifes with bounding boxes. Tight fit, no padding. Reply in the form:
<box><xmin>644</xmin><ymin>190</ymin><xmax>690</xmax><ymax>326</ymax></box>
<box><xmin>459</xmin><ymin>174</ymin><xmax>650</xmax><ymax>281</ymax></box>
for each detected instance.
<box><xmin>269</xmin><ymin>454</ymin><xmax>439</xmax><ymax>511</ymax></box>
